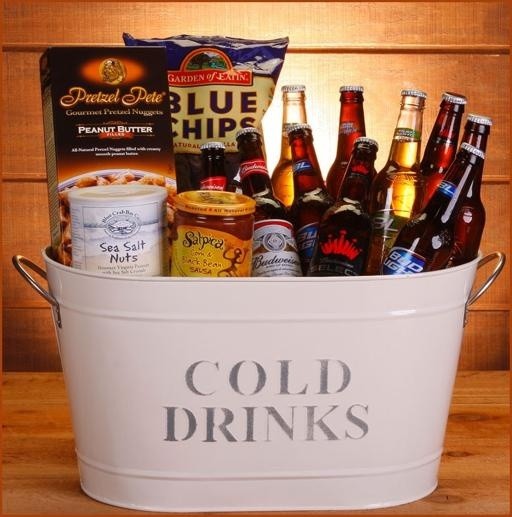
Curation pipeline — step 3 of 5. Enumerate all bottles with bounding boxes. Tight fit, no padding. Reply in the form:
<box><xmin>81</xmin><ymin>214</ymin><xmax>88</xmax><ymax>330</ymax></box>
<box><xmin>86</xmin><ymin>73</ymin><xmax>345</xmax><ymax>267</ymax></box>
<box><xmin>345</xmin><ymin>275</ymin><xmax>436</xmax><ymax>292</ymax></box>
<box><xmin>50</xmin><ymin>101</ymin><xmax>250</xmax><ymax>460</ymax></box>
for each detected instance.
<box><xmin>305</xmin><ymin>137</ymin><xmax>379</xmax><ymax>276</ymax></box>
<box><xmin>378</xmin><ymin>143</ymin><xmax>487</xmax><ymax>279</ymax></box>
<box><xmin>366</xmin><ymin>89</ymin><xmax>424</xmax><ymax>275</ymax></box>
<box><xmin>448</xmin><ymin>114</ymin><xmax>493</xmax><ymax>269</ymax></box>
<box><xmin>287</xmin><ymin>125</ymin><xmax>336</xmax><ymax>277</ymax></box>
<box><xmin>326</xmin><ymin>85</ymin><xmax>369</xmax><ymax>204</ymax></box>
<box><xmin>270</xmin><ymin>86</ymin><xmax>311</xmax><ymax>212</ymax></box>
<box><xmin>422</xmin><ymin>91</ymin><xmax>467</xmax><ymax>214</ymax></box>
<box><xmin>200</xmin><ymin>142</ymin><xmax>227</xmax><ymax>192</ymax></box>
<box><xmin>236</xmin><ymin>127</ymin><xmax>303</xmax><ymax>276</ymax></box>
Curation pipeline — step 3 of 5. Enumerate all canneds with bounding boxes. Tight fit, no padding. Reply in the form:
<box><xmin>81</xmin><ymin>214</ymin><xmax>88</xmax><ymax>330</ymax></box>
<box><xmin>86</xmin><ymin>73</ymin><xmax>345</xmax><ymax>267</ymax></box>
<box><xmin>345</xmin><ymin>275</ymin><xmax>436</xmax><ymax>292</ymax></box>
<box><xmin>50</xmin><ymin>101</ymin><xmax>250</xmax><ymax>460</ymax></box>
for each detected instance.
<box><xmin>171</xmin><ymin>191</ymin><xmax>256</xmax><ymax>277</ymax></box>
<box><xmin>67</xmin><ymin>185</ymin><xmax>167</xmax><ymax>279</ymax></box>
